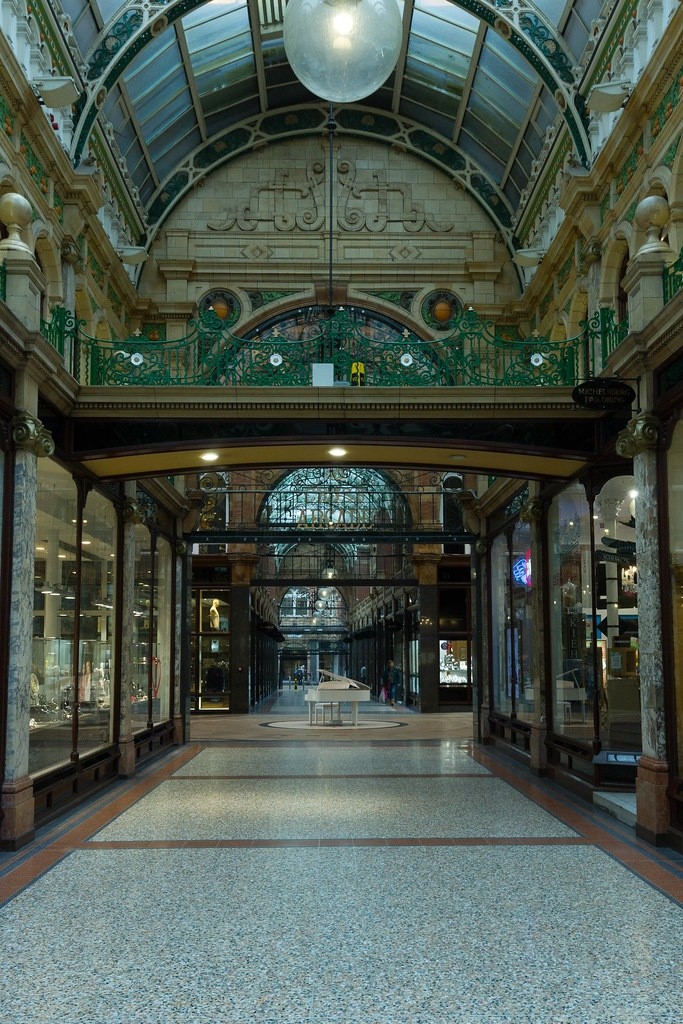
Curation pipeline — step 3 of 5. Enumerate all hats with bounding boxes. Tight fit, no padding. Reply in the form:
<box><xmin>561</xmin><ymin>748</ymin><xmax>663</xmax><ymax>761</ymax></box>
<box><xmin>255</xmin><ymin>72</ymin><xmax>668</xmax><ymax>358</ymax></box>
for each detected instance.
<box><xmin>387</xmin><ymin>660</ymin><xmax>394</xmax><ymax>666</ymax></box>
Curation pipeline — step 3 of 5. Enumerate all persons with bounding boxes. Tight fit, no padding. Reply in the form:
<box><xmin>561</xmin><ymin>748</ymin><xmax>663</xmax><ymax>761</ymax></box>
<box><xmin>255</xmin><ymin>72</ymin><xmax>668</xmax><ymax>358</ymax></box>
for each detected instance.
<box><xmin>361</xmin><ymin>664</ymin><xmax>367</xmax><ymax>684</ymax></box>
<box><xmin>382</xmin><ymin>660</ymin><xmax>400</xmax><ymax>706</ymax></box>
<box><xmin>210</xmin><ymin>599</ymin><xmax>219</xmax><ymax>627</ymax></box>
<box><xmin>294</xmin><ymin>664</ymin><xmax>306</xmax><ymax>684</ymax></box>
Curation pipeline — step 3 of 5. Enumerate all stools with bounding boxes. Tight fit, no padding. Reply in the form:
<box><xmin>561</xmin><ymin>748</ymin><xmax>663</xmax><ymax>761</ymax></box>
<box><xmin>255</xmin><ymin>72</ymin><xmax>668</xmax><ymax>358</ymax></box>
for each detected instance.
<box><xmin>314</xmin><ymin>703</ymin><xmax>341</xmax><ymax>725</ymax></box>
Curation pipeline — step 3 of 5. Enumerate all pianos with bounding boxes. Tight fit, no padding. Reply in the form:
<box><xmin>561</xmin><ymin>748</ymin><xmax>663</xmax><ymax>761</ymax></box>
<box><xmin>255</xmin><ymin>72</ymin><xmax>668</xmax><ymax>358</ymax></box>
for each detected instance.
<box><xmin>304</xmin><ymin>668</ymin><xmax>372</xmax><ymax>726</ymax></box>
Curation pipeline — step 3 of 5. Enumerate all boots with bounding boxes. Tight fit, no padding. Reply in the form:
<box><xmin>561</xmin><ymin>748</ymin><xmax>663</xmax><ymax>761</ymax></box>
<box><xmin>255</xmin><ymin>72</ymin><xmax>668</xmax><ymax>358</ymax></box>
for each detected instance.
<box><xmin>391</xmin><ymin>699</ymin><xmax>395</xmax><ymax>706</ymax></box>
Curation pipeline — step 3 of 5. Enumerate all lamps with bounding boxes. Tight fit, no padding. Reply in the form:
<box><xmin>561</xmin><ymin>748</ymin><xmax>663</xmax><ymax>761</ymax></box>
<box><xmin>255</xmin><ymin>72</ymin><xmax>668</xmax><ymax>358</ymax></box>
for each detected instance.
<box><xmin>282</xmin><ymin>0</ymin><xmax>402</xmax><ymax>103</ymax></box>
<box><xmin>556</xmin><ymin>701</ymin><xmax>573</xmax><ymax>726</ymax></box>
<box><xmin>512</xmin><ymin>248</ymin><xmax>547</xmax><ymax>267</ymax></box>
<box><xmin>584</xmin><ymin>78</ymin><xmax>637</xmax><ymax>113</ymax></box>
<box><xmin>113</xmin><ymin>245</ymin><xmax>150</xmax><ymax>266</ymax></box>
<box><xmin>28</xmin><ymin>76</ymin><xmax>82</xmax><ymax>109</ymax></box>
<box><xmin>321</xmin><ymin>567</ymin><xmax>338</xmax><ymax>579</ymax></box>
<box><xmin>314</xmin><ymin>600</ymin><xmax>327</xmax><ymax>612</ymax></box>
<box><xmin>318</xmin><ymin>587</ymin><xmax>331</xmax><ymax>600</ymax></box>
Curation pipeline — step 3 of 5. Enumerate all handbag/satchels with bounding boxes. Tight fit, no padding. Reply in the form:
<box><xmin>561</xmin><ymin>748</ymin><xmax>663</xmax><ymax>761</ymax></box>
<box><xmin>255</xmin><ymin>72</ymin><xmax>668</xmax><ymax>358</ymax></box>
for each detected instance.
<box><xmin>379</xmin><ymin>687</ymin><xmax>388</xmax><ymax>703</ymax></box>
